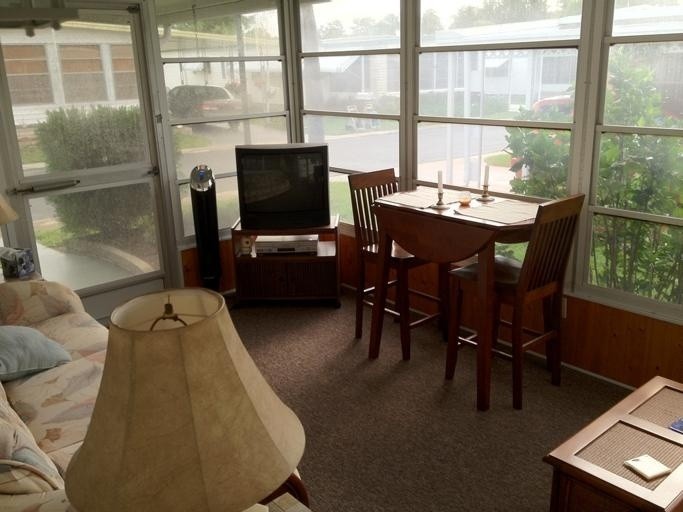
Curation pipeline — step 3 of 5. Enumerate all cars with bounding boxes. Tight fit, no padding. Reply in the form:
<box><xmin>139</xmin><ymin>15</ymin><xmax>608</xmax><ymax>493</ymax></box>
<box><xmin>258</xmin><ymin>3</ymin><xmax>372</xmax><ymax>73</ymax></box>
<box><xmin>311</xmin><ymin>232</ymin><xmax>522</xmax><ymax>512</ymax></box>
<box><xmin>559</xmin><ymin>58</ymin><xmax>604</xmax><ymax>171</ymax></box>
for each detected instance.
<box><xmin>168</xmin><ymin>85</ymin><xmax>243</xmax><ymax>129</ymax></box>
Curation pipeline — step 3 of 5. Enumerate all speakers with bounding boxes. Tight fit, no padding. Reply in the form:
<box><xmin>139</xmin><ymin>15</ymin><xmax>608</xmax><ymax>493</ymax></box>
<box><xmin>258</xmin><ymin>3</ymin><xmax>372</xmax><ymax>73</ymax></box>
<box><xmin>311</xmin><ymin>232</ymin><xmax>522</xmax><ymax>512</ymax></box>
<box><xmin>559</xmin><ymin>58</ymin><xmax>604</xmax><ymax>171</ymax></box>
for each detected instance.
<box><xmin>191</xmin><ymin>165</ymin><xmax>222</xmax><ymax>292</ymax></box>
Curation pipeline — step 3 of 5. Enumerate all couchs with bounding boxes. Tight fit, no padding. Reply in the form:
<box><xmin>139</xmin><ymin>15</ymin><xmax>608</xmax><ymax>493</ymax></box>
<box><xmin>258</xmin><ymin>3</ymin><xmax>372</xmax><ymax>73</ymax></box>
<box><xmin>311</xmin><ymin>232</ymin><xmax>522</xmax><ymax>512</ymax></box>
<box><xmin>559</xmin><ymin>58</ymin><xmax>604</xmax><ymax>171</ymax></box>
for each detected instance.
<box><xmin>0</xmin><ymin>280</ymin><xmax>307</xmax><ymax>512</ymax></box>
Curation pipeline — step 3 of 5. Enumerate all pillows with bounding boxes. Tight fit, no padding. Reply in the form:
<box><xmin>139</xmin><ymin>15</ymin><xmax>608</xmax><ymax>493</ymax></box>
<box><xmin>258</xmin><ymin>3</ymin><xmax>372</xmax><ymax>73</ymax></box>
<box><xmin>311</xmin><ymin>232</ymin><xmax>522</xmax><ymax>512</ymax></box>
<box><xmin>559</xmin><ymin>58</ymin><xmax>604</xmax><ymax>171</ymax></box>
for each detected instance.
<box><xmin>0</xmin><ymin>323</ymin><xmax>71</xmax><ymax>380</ymax></box>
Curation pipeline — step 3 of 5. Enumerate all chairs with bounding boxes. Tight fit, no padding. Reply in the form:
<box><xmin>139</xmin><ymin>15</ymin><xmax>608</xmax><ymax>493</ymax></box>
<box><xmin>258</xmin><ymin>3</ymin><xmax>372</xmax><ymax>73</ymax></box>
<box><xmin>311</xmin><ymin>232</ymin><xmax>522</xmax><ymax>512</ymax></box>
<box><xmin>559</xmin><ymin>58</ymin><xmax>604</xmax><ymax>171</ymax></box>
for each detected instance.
<box><xmin>349</xmin><ymin>169</ymin><xmax>449</xmax><ymax>363</ymax></box>
<box><xmin>442</xmin><ymin>191</ymin><xmax>585</xmax><ymax>410</ymax></box>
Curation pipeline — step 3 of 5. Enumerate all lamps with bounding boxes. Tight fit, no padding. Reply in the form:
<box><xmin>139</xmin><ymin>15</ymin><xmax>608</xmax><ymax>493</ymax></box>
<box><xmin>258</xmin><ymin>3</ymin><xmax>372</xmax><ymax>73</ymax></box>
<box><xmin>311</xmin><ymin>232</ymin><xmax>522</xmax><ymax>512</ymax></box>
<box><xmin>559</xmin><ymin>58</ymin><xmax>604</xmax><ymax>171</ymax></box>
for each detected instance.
<box><xmin>1</xmin><ymin>193</ymin><xmax>20</xmax><ymax>225</ymax></box>
<box><xmin>64</xmin><ymin>288</ymin><xmax>306</xmax><ymax>512</ymax></box>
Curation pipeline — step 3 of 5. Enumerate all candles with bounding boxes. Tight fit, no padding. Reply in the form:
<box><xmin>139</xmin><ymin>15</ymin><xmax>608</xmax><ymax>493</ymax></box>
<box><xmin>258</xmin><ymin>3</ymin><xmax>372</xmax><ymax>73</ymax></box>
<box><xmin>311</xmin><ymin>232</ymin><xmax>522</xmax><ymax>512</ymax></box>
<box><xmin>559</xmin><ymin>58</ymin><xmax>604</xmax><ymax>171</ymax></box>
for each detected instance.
<box><xmin>478</xmin><ymin>164</ymin><xmax>495</xmax><ymax>201</ymax></box>
<box><xmin>429</xmin><ymin>169</ymin><xmax>451</xmax><ymax>209</ymax></box>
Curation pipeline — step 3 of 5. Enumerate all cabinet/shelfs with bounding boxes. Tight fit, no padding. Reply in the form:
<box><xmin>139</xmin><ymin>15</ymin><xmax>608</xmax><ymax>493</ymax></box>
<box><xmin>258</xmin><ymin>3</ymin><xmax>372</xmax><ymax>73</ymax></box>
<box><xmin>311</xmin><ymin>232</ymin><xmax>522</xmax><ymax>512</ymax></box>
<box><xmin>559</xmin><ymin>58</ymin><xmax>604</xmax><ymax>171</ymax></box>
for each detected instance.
<box><xmin>541</xmin><ymin>375</ymin><xmax>683</xmax><ymax>512</ymax></box>
<box><xmin>230</xmin><ymin>213</ymin><xmax>342</xmax><ymax>308</ymax></box>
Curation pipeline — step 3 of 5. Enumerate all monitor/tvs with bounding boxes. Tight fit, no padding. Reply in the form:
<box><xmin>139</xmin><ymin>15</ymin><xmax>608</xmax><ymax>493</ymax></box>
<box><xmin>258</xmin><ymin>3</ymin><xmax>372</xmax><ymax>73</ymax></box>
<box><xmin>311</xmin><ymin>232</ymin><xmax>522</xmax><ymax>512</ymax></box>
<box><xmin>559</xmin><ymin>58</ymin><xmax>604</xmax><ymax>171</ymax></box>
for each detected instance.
<box><xmin>234</xmin><ymin>142</ymin><xmax>332</xmax><ymax>230</ymax></box>
